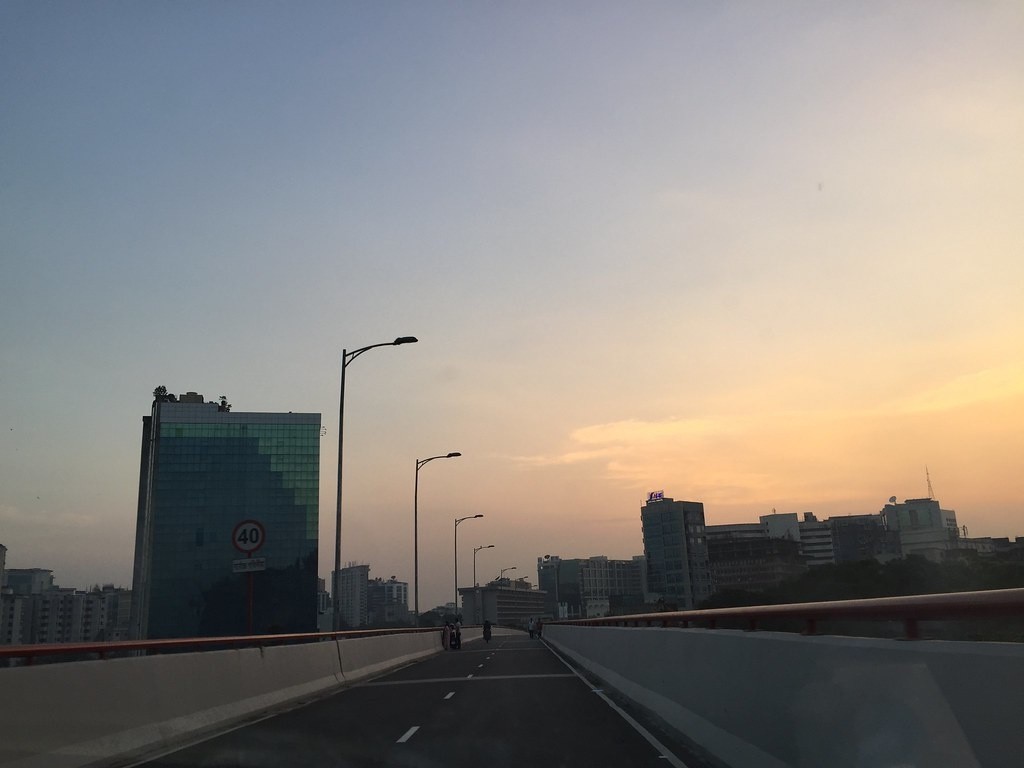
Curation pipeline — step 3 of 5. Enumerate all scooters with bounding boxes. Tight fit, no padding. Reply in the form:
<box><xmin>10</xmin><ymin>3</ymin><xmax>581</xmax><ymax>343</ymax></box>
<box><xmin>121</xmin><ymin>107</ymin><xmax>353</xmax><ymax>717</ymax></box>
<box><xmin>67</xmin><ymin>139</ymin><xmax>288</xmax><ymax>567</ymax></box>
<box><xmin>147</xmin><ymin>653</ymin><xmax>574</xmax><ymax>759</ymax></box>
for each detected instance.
<box><xmin>484</xmin><ymin>626</ymin><xmax>491</xmax><ymax>644</ymax></box>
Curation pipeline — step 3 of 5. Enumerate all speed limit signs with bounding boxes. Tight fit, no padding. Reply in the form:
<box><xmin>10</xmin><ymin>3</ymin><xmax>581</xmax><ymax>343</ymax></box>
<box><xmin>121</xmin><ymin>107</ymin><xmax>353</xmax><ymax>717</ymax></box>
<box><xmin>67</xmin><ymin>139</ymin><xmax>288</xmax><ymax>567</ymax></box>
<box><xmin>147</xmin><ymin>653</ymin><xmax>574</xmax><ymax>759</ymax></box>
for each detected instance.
<box><xmin>233</xmin><ymin>519</ymin><xmax>265</xmax><ymax>553</ymax></box>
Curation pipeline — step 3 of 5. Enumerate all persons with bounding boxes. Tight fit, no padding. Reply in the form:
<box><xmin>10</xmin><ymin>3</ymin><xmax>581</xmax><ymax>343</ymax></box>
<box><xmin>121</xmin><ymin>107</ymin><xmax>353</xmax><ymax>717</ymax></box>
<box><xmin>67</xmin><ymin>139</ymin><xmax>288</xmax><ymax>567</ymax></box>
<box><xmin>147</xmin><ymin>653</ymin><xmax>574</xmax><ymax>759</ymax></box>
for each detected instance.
<box><xmin>483</xmin><ymin>619</ymin><xmax>492</xmax><ymax>640</ymax></box>
<box><xmin>443</xmin><ymin>618</ymin><xmax>462</xmax><ymax>650</ymax></box>
<box><xmin>528</xmin><ymin>618</ymin><xmax>542</xmax><ymax>639</ymax></box>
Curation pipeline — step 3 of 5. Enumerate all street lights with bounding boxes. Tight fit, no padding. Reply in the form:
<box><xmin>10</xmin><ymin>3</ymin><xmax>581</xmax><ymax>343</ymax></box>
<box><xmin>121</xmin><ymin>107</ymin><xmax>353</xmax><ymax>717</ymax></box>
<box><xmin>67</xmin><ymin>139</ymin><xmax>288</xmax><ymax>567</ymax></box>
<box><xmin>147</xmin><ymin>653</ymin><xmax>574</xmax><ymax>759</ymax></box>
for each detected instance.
<box><xmin>455</xmin><ymin>515</ymin><xmax>483</xmax><ymax>618</ymax></box>
<box><xmin>501</xmin><ymin>567</ymin><xmax>537</xmax><ymax>631</ymax></box>
<box><xmin>414</xmin><ymin>453</ymin><xmax>461</xmax><ymax>629</ymax></box>
<box><xmin>331</xmin><ymin>337</ymin><xmax>418</xmax><ymax>641</ymax></box>
<box><xmin>474</xmin><ymin>545</ymin><xmax>494</xmax><ymax>627</ymax></box>
